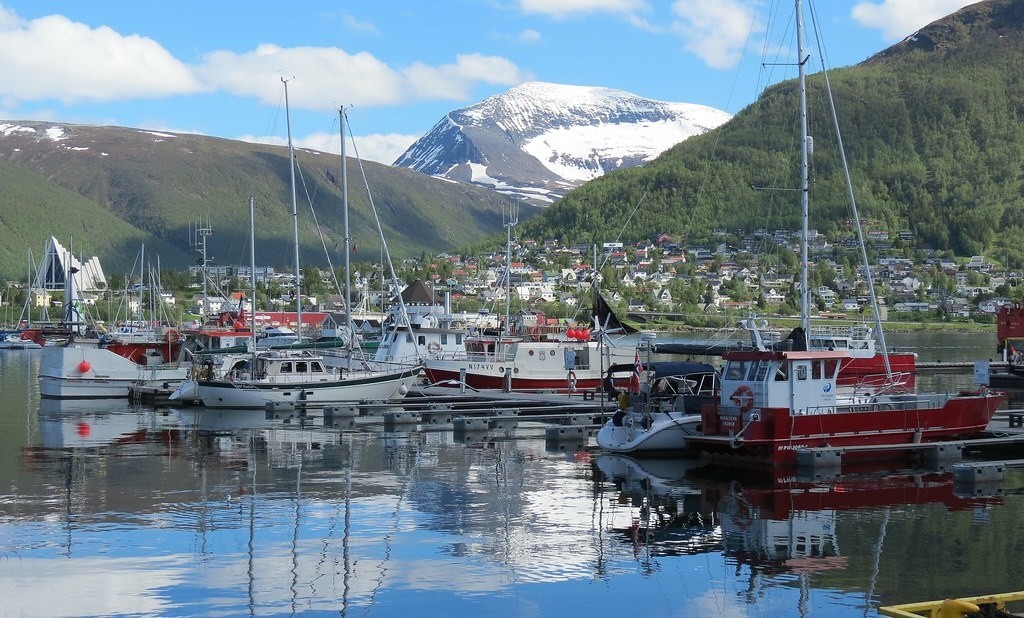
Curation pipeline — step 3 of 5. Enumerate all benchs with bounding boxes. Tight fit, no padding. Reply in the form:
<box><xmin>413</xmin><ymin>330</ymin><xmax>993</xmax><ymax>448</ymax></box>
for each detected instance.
<box><xmin>583</xmin><ymin>389</ymin><xmax>613</xmax><ymax>401</ymax></box>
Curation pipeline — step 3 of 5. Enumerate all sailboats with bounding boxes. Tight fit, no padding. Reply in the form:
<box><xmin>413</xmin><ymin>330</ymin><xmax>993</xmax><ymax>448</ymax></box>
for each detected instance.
<box><xmin>21</xmin><ymin>394</ymin><xmax>593</xmax><ymax>618</ymax></box>
<box><xmin>0</xmin><ymin>0</ymin><xmax>1024</xmax><ymax>464</ymax></box>
<box><xmin>591</xmin><ymin>451</ymin><xmax>697</xmax><ymax>576</ymax></box>
<box><xmin>713</xmin><ymin>469</ymin><xmax>1006</xmax><ymax>618</ymax></box>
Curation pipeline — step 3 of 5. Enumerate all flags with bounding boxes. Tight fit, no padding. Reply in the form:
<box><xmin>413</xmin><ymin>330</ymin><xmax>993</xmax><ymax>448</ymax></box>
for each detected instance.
<box><xmin>237</xmin><ymin>296</ymin><xmax>244</xmax><ymax>325</ymax></box>
<box><xmin>633</xmin><ymin>352</ymin><xmax>643</xmax><ymax>379</ymax></box>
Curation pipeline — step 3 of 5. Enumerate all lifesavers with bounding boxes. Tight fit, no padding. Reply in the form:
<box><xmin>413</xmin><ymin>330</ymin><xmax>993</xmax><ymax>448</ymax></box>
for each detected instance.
<box><xmin>734</xmin><ymin>386</ymin><xmax>754</xmax><ymax>411</ymax></box>
<box><xmin>166</xmin><ymin>329</ymin><xmax>179</xmax><ymax>344</ymax></box>
<box><xmin>530</xmin><ymin>325</ymin><xmax>541</xmax><ymax>340</ymax></box>
<box><xmin>428</xmin><ymin>342</ymin><xmax>441</xmax><ymax>354</ymax></box>
<box><xmin>567</xmin><ymin>371</ymin><xmax>578</xmax><ymax>387</ymax></box>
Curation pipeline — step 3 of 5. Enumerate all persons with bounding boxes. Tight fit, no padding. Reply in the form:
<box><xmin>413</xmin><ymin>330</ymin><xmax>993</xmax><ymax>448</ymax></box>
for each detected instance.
<box><xmin>1010</xmin><ymin>350</ymin><xmax>1023</xmax><ymax>364</ymax></box>
<box><xmin>604</xmin><ymin>372</ymin><xmax>622</xmax><ymax>401</ymax></box>
<box><xmin>240</xmin><ymin>368</ymin><xmax>250</xmax><ymax>381</ymax></box>
